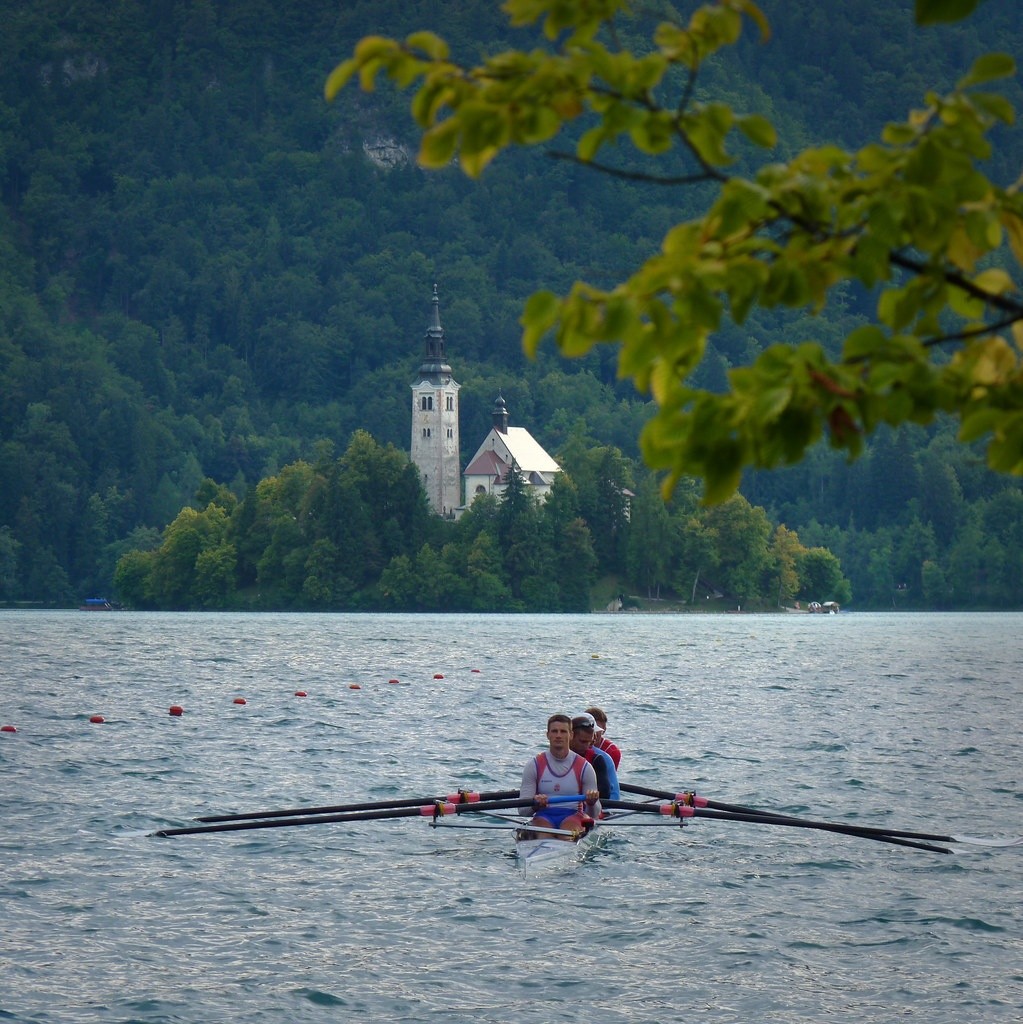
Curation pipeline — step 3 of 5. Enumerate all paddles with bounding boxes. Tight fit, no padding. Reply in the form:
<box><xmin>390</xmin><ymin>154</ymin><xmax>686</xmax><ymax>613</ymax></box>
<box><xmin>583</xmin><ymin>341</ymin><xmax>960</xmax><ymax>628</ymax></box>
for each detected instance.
<box><xmin>619</xmin><ymin>780</ymin><xmax>973</xmax><ymax>856</ymax></box>
<box><xmin>151</xmin><ymin>792</ymin><xmax>589</xmax><ymax>837</ymax></box>
<box><xmin>191</xmin><ymin>786</ymin><xmax>522</xmax><ymax>825</ymax></box>
<box><xmin>600</xmin><ymin>798</ymin><xmax>1023</xmax><ymax>849</ymax></box>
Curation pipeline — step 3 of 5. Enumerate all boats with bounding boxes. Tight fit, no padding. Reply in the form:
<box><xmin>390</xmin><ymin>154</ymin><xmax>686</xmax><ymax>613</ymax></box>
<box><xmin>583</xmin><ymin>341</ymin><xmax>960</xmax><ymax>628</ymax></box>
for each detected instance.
<box><xmin>512</xmin><ymin>838</ymin><xmax>578</xmax><ymax>877</ymax></box>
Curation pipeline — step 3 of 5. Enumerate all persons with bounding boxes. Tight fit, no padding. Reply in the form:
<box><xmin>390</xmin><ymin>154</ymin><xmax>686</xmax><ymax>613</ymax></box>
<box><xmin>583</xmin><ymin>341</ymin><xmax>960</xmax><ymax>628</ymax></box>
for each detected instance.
<box><xmin>573</xmin><ymin>713</ymin><xmax>621</xmax><ymax>801</ymax></box>
<box><xmin>518</xmin><ymin>715</ymin><xmax>602</xmax><ymax>842</ymax></box>
<box><xmin>567</xmin><ymin>717</ymin><xmax>611</xmax><ymax>800</ymax></box>
<box><xmin>585</xmin><ymin>707</ymin><xmax>621</xmax><ymax>772</ymax></box>
